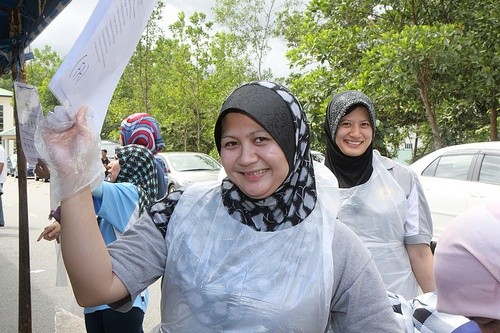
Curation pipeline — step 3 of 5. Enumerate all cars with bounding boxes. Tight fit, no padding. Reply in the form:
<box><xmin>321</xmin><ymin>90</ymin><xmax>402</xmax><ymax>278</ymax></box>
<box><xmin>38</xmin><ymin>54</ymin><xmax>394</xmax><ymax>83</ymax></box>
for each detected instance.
<box><xmin>407</xmin><ymin>141</ymin><xmax>500</xmax><ymax>242</ymax></box>
<box><xmin>100</xmin><ymin>141</ymin><xmax>124</xmax><ymax>163</ymax></box>
<box><xmin>219</xmin><ymin>150</ymin><xmax>326</xmax><ymax>182</ymax></box>
<box><xmin>7</xmin><ymin>154</ymin><xmax>48</xmax><ymax>182</ymax></box>
<box><xmin>154</xmin><ymin>152</ymin><xmax>227</xmax><ymax>186</ymax></box>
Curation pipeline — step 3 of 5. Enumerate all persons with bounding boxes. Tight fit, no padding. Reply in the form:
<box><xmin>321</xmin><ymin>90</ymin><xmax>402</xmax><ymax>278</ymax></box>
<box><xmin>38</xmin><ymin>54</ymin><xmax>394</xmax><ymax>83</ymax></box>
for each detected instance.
<box><xmin>41</xmin><ymin>80</ymin><xmax>402</xmax><ymax>333</ymax></box>
<box><xmin>36</xmin><ymin>144</ymin><xmax>158</xmax><ymax>333</ymax></box>
<box><xmin>318</xmin><ymin>91</ymin><xmax>435</xmax><ymax>333</ymax></box>
<box><xmin>100</xmin><ymin>111</ymin><xmax>168</xmax><ymax>204</ymax></box>
<box><xmin>434</xmin><ymin>201</ymin><xmax>500</xmax><ymax>333</ymax></box>
<box><xmin>0</xmin><ymin>144</ymin><xmax>7</xmax><ymax>228</ymax></box>
